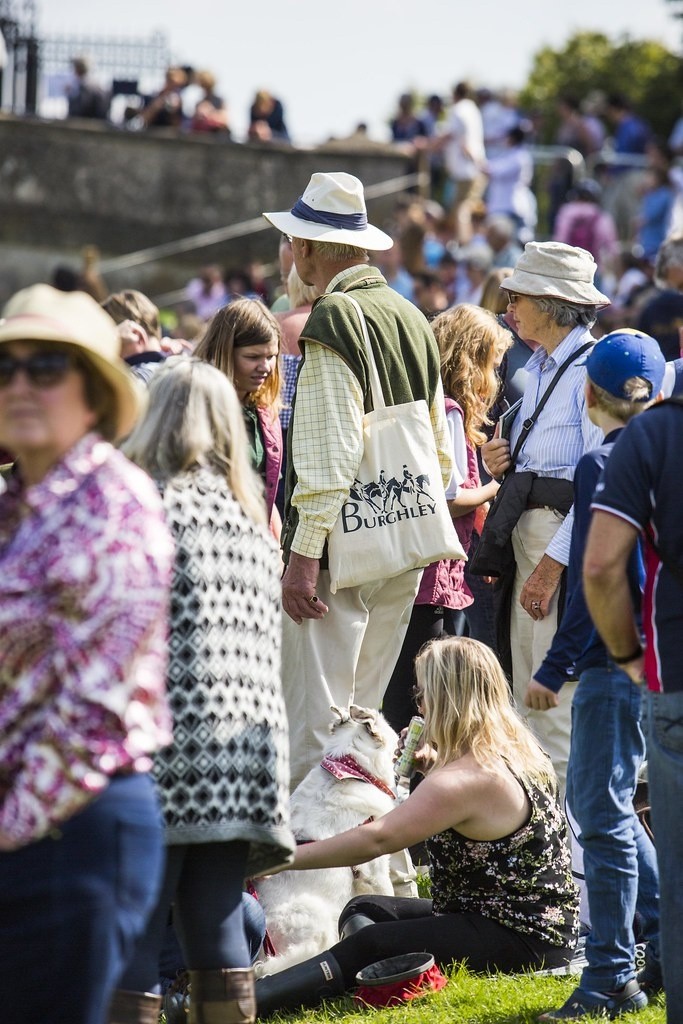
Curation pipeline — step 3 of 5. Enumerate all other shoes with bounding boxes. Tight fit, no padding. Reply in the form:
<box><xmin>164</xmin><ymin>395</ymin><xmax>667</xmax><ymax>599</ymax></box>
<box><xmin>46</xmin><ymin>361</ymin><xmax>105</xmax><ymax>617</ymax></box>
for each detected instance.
<box><xmin>536</xmin><ymin>976</ymin><xmax>648</xmax><ymax>1023</ymax></box>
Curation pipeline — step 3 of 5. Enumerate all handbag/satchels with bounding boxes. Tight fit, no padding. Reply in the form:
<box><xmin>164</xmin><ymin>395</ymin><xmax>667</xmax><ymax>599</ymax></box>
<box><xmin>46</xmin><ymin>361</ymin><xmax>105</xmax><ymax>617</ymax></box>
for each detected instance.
<box><xmin>325</xmin><ymin>289</ymin><xmax>469</xmax><ymax>596</ymax></box>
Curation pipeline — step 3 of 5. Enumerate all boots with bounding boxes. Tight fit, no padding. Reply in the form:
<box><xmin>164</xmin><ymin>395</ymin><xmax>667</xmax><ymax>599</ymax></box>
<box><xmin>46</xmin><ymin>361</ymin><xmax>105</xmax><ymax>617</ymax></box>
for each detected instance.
<box><xmin>338</xmin><ymin>913</ymin><xmax>377</xmax><ymax>941</ymax></box>
<box><xmin>107</xmin><ymin>987</ymin><xmax>162</xmax><ymax>1023</ymax></box>
<box><xmin>186</xmin><ymin>967</ymin><xmax>260</xmax><ymax>1023</ymax></box>
<box><xmin>255</xmin><ymin>951</ymin><xmax>344</xmax><ymax>1017</ymax></box>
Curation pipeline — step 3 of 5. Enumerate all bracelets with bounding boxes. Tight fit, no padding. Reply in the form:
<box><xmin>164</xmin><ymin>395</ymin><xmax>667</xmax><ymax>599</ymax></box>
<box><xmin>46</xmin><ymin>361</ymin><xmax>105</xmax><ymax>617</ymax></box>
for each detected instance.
<box><xmin>608</xmin><ymin>649</ymin><xmax>642</xmax><ymax>666</ymax></box>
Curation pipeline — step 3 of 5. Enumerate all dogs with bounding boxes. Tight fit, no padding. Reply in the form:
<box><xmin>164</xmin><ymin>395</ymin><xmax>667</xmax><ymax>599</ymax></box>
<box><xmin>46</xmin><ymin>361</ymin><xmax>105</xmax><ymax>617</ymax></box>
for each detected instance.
<box><xmin>245</xmin><ymin>704</ymin><xmax>423</xmax><ymax>982</ymax></box>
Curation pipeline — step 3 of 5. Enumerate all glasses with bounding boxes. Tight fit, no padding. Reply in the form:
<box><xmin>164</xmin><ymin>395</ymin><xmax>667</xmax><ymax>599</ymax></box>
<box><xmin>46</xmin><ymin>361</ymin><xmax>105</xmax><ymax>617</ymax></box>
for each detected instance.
<box><xmin>0</xmin><ymin>350</ymin><xmax>84</xmax><ymax>391</ymax></box>
<box><xmin>507</xmin><ymin>291</ymin><xmax>520</xmax><ymax>304</ymax></box>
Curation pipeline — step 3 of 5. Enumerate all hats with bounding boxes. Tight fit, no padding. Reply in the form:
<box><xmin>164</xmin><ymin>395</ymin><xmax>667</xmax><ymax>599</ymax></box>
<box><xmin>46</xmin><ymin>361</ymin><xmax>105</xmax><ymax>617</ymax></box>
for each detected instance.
<box><xmin>574</xmin><ymin>328</ymin><xmax>666</xmax><ymax>402</ymax></box>
<box><xmin>0</xmin><ymin>282</ymin><xmax>150</xmax><ymax>448</ymax></box>
<box><xmin>262</xmin><ymin>171</ymin><xmax>394</xmax><ymax>251</ymax></box>
<box><xmin>500</xmin><ymin>240</ymin><xmax>611</xmax><ymax>311</ymax></box>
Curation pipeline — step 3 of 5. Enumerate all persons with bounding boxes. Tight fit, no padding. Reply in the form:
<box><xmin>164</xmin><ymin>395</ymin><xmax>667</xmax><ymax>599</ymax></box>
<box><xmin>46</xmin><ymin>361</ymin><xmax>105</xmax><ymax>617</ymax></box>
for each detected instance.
<box><xmin>129</xmin><ymin>354</ymin><xmax>289</xmax><ymax>1024</ymax></box>
<box><xmin>261</xmin><ymin>171</ymin><xmax>454</xmax><ymax>801</ymax></box>
<box><xmin>528</xmin><ymin>327</ymin><xmax>663</xmax><ymax>1017</ymax></box>
<box><xmin>0</xmin><ymin>285</ymin><xmax>160</xmax><ymax>1024</ymax></box>
<box><xmin>40</xmin><ymin>57</ymin><xmax>683</xmax><ymax>788</ymax></box>
<box><xmin>165</xmin><ymin>635</ymin><xmax>577</xmax><ymax>1024</ymax></box>
<box><xmin>465</xmin><ymin>241</ymin><xmax>618</xmax><ymax>793</ymax></box>
<box><xmin>582</xmin><ymin>364</ymin><xmax>681</xmax><ymax>1020</ymax></box>
